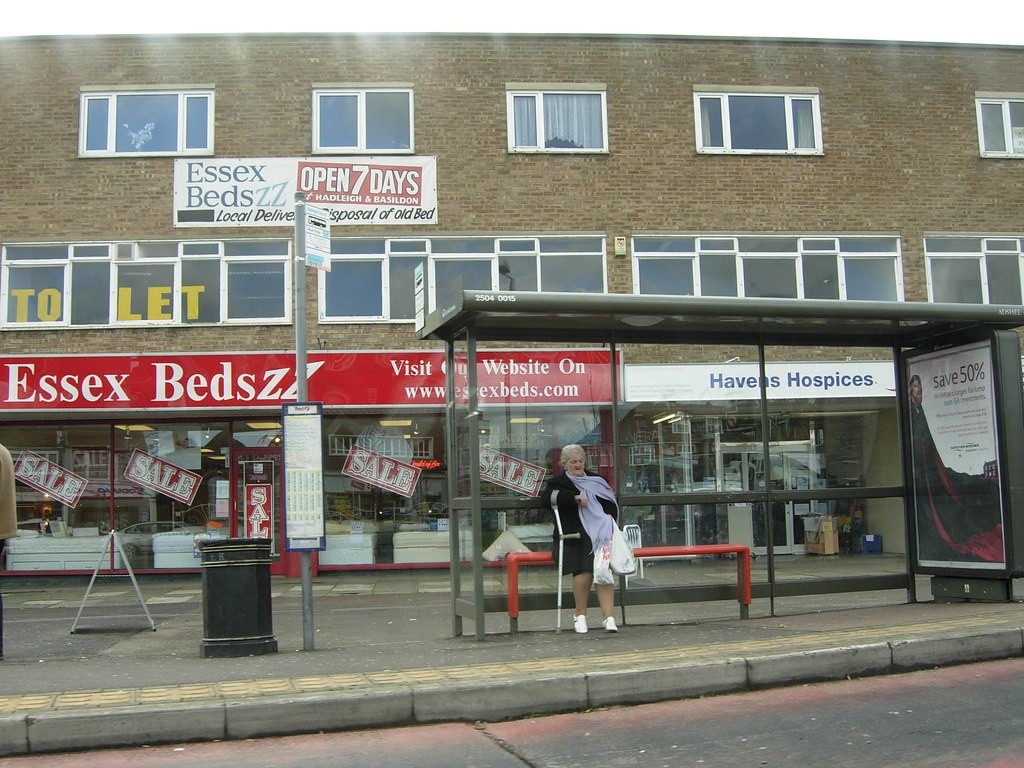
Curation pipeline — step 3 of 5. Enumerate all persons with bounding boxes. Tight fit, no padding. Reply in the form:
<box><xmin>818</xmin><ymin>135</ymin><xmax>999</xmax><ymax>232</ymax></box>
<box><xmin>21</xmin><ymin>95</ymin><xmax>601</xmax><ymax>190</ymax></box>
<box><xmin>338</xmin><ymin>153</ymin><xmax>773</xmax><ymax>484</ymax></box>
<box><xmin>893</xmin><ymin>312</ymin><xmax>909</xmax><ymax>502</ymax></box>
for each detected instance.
<box><xmin>542</xmin><ymin>444</ymin><xmax>618</xmax><ymax>634</ymax></box>
<box><xmin>0</xmin><ymin>443</ymin><xmax>18</xmax><ymax>661</ymax></box>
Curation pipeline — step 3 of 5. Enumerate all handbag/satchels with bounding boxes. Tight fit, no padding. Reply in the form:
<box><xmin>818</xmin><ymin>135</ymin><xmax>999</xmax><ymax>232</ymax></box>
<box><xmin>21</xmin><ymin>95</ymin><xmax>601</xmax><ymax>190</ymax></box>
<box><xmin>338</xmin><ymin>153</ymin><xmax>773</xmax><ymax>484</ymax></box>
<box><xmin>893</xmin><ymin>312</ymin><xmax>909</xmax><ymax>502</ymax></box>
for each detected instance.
<box><xmin>609</xmin><ymin>517</ymin><xmax>636</xmax><ymax>575</ymax></box>
<box><xmin>593</xmin><ymin>545</ymin><xmax>615</xmax><ymax>585</ymax></box>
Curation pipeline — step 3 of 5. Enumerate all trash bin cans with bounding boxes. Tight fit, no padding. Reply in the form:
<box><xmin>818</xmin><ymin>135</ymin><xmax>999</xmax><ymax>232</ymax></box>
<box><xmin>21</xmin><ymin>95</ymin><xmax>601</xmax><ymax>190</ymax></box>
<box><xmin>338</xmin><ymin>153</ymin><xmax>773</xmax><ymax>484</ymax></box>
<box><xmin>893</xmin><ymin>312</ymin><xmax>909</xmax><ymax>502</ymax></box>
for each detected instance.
<box><xmin>199</xmin><ymin>538</ymin><xmax>279</xmax><ymax>658</ymax></box>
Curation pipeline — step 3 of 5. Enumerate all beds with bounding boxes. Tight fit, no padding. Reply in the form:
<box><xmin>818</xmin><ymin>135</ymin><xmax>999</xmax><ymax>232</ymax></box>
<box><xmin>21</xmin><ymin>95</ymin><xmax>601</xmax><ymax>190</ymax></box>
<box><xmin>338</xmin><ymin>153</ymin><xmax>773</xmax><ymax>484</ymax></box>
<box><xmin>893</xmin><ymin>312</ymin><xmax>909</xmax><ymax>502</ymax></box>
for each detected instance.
<box><xmin>151</xmin><ymin>503</ymin><xmax>243</xmax><ymax>569</ymax></box>
<box><xmin>310</xmin><ymin>506</ymin><xmax>379</xmax><ymax>564</ymax></box>
<box><xmin>392</xmin><ymin>501</ymin><xmax>474</xmax><ymax>564</ymax></box>
<box><xmin>4</xmin><ymin>518</ymin><xmax>122</xmax><ymax>569</ymax></box>
<box><xmin>497</xmin><ymin>512</ymin><xmax>554</xmax><ymax>543</ymax></box>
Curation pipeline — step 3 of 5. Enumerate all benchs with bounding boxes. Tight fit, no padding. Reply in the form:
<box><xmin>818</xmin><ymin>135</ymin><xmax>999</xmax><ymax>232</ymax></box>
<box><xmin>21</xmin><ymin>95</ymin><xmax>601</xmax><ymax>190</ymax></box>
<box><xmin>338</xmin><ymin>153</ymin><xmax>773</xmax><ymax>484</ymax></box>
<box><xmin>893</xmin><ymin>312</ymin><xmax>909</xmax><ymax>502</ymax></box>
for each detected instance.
<box><xmin>507</xmin><ymin>543</ymin><xmax>752</xmax><ymax>634</ymax></box>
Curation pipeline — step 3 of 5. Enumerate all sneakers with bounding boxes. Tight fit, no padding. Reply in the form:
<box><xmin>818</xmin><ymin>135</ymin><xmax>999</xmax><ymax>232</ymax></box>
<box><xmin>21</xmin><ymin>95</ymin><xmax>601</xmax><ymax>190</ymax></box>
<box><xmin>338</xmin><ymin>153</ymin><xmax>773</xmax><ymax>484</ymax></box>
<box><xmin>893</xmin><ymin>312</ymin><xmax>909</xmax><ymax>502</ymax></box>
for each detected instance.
<box><xmin>602</xmin><ymin>616</ymin><xmax>618</xmax><ymax>632</ymax></box>
<box><xmin>573</xmin><ymin>613</ymin><xmax>588</xmax><ymax>634</ymax></box>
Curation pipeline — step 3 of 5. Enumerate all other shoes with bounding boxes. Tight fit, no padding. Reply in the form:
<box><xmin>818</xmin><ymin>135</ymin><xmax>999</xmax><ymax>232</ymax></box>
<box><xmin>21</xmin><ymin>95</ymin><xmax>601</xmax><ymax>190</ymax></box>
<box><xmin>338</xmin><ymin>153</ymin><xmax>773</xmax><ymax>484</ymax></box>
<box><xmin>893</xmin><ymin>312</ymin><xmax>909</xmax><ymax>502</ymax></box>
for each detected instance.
<box><xmin>0</xmin><ymin>654</ymin><xmax>5</xmax><ymax>661</ymax></box>
